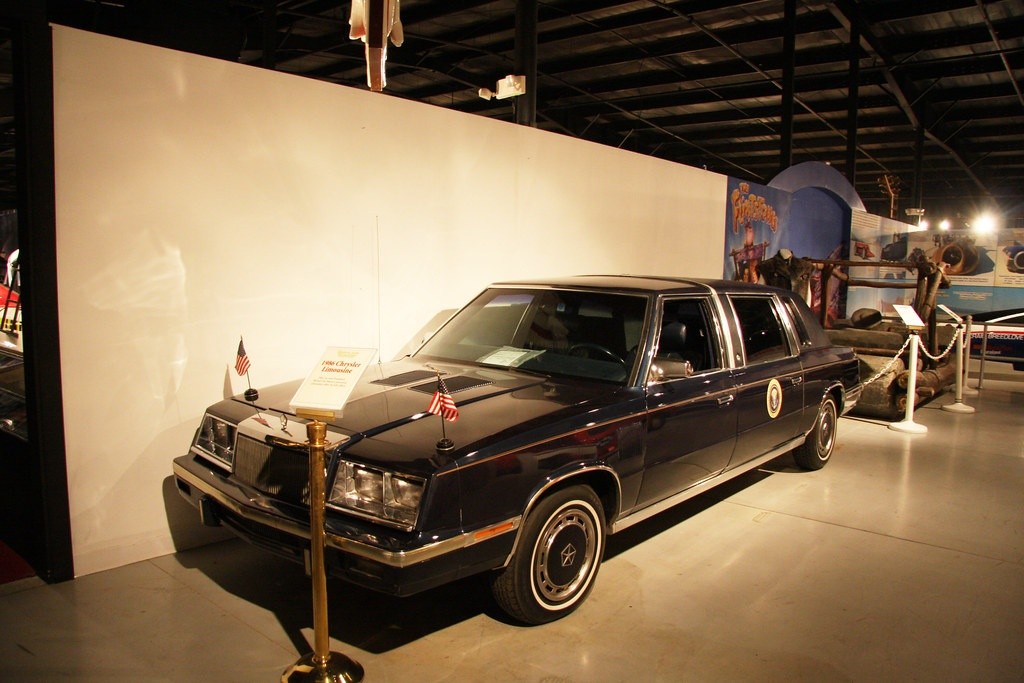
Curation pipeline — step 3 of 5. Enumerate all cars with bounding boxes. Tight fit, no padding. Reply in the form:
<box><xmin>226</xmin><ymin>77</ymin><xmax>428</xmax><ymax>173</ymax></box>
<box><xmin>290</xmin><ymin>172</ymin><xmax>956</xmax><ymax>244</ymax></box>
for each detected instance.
<box><xmin>171</xmin><ymin>273</ymin><xmax>866</xmax><ymax>625</ymax></box>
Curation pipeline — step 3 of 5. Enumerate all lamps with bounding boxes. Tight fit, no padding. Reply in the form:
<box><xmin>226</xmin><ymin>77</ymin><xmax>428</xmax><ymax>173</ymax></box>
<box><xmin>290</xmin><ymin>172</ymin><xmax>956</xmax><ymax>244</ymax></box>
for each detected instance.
<box><xmin>477</xmin><ymin>74</ymin><xmax>527</xmax><ymax>101</ymax></box>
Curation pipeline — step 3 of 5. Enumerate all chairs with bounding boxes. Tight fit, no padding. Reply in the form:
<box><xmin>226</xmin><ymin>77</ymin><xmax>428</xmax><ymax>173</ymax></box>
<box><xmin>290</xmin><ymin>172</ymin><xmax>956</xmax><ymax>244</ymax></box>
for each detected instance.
<box><xmin>657</xmin><ymin>321</ymin><xmax>704</xmax><ymax>369</ymax></box>
